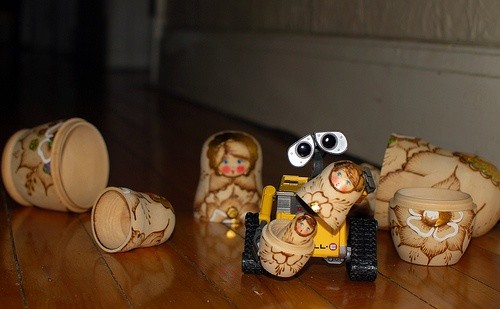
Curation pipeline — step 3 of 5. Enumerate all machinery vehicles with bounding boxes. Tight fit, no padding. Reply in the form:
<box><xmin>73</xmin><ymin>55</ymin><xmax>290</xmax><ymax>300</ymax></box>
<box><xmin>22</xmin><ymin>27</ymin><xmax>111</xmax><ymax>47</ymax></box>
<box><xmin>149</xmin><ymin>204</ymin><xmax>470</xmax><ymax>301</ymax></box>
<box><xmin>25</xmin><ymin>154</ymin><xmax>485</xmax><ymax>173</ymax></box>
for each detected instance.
<box><xmin>241</xmin><ymin>129</ymin><xmax>379</xmax><ymax>281</ymax></box>
